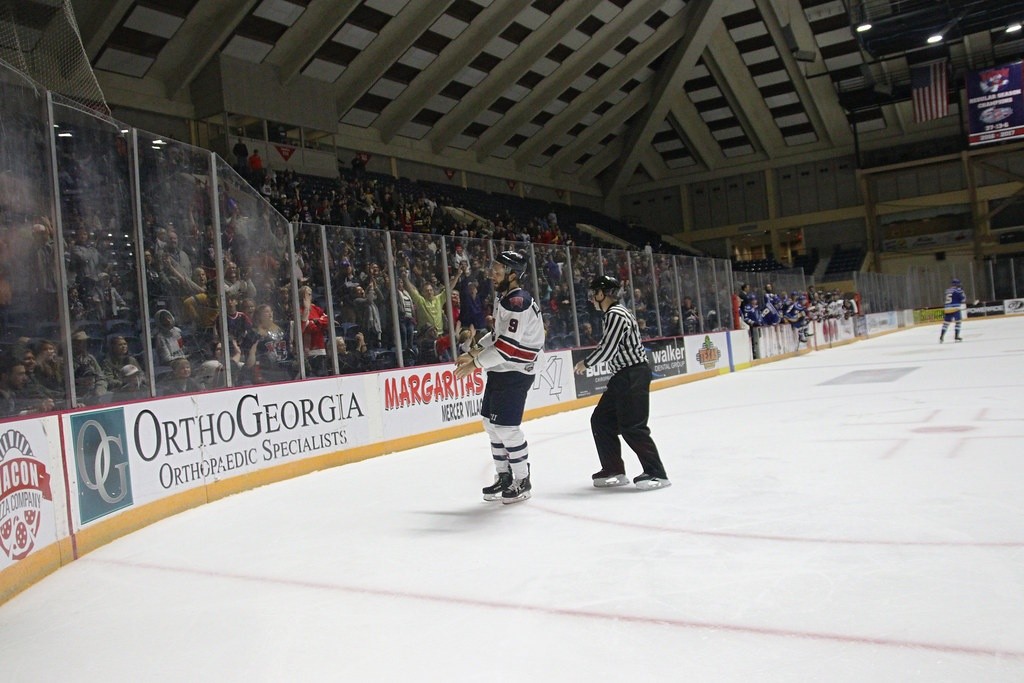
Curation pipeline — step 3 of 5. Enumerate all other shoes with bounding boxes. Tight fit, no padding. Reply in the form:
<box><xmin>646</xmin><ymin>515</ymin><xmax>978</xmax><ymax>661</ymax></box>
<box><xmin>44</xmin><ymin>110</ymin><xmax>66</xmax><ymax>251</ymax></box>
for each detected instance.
<box><xmin>798</xmin><ymin>328</ymin><xmax>814</xmax><ymax>343</ymax></box>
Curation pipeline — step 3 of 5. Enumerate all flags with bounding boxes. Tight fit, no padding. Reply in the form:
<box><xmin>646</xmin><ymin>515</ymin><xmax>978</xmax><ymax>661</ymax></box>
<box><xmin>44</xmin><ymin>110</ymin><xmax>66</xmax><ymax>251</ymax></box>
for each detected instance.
<box><xmin>910</xmin><ymin>61</ymin><xmax>950</xmax><ymax>124</ymax></box>
<box><xmin>965</xmin><ymin>64</ymin><xmax>1024</xmax><ymax>145</ymax></box>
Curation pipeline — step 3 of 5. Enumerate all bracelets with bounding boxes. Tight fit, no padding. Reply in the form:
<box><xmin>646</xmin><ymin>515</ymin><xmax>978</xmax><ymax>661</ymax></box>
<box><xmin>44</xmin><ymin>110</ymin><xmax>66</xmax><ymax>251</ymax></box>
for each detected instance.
<box><xmin>468</xmin><ymin>351</ymin><xmax>474</xmax><ymax>359</ymax></box>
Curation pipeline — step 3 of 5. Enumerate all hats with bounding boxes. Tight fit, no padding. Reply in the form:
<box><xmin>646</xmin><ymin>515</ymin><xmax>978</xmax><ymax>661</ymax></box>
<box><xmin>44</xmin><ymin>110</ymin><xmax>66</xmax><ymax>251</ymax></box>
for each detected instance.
<box><xmin>32</xmin><ymin>223</ymin><xmax>47</xmax><ymax>234</ymax></box>
<box><xmin>98</xmin><ymin>273</ymin><xmax>110</xmax><ymax>280</ymax></box>
<box><xmin>72</xmin><ymin>331</ymin><xmax>91</xmax><ymax>341</ymax></box>
<box><xmin>280</xmin><ymin>194</ymin><xmax>287</xmax><ymax>199</ymax></box>
<box><xmin>120</xmin><ymin>364</ymin><xmax>140</xmax><ymax>377</ymax></box>
<box><xmin>951</xmin><ymin>279</ymin><xmax>960</xmax><ymax>286</ymax></box>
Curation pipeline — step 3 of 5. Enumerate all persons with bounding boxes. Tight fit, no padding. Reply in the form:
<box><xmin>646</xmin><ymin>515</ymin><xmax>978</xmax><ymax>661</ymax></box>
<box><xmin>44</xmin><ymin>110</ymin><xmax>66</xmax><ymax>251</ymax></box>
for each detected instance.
<box><xmin>217</xmin><ymin>293</ymin><xmax>252</xmax><ymax>343</ymax></box>
<box><xmin>452</xmin><ymin>250</ymin><xmax>546</xmax><ymax>504</ymax></box>
<box><xmin>68</xmin><ymin>330</ymin><xmax>107</xmax><ymax>400</ymax></box>
<box><xmin>352</xmin><ymin>274</ymin><xmax>384</xmax><ymax>349</ymax></box>
<box><xmin>1</xmin><ymin>90</ymin><xmax>738</xmax><ymax>417</ymax></box>
<box><xmin>462</xmin><ymin>270</ymin><xmax>492</xmax><ymax>330</ymax></box>
<box><xmin>400</xmin><ymin>269</ymin><xmax>463</xmax><ymax>360</ymax></box>
<box><xmin>157</xmin><ymin>309</ymin><xmax>188</xmax><ymax>365</ymax></box>
<box><xmin>295</xmin><ymin>283</ymin><xmax>331</xmax><ymax>377</ymax></box>
<box><xmin>739</xmin><ymin>284</ymin><xmax>864</xmax><ymax>345</ymax></box>
<box><xmin>161</xmin><ymin>231</ymin><xmax>192</xmax><ymax>282</ymax></box>
<box><xmin>574</xmin><ymin>276</ymin><xmax>671</xmax><ymax>490</ymax></box>
<box><xmin>244</xmin><ymin>304</ymin><xmax>291</xmax><ymax>366</ymax></box>
<box><xmin>940</xmin><ymin>279</ymin><xmax>967</xmax><ymax>340</ymax></box>
<box><xmin>248</xmin><ymin>149</ymin><xmax>263</xmax><ymax>185</ymax></box>
<box><xmin>112</xmin><ymin>336</ymin><xmax>147</xmax><ymax>390</ymax></box>
<box><xmin>234</xmin><ymin>139</ymin><xmax>247</xmax><ymax>167</ymax></box>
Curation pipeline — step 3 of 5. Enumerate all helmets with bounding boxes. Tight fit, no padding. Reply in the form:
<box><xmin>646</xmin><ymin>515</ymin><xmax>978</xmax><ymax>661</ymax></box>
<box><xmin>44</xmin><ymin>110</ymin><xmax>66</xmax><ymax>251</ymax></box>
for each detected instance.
<box><xmin>495</xmin><ymin>250</ymin><xmax>528</xmax><ymax>272</ymax></box>
<box><xmin>588</xmin><ymin>276</ymin><xmax>620</xmax><ymax>290</ymax></box>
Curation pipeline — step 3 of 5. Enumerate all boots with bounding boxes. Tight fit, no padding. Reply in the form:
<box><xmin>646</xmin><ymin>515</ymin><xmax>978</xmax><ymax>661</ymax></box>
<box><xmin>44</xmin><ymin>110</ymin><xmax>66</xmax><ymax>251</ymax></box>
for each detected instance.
<box><xmin>482</xmin><ymin>464</ymin><xmax>513</xmax><ymax>501</ymax></box>
<box><xmin>500</xmin><ymin>463</ymin><xmax>532</xmax><ymax>505</ymax></box>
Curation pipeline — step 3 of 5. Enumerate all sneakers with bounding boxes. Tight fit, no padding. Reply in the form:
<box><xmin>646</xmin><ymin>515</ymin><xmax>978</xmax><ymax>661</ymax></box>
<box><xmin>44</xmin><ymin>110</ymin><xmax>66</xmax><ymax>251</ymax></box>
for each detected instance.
<box><xmin>955</xmin><ymin>337</ymin><xmax>962</xmax><ymax>341</ymax></box>
<box><xmin>592</xmin><ymin>468</ymin><xmax>630</xmax><ymax>488</ymax></box>
<box><xmin>939</xmin><ymin>337</ymin><xmax>943</xmax><ymax>343</ymax></box>
<box><xmin>633</xmin><ymin>472</ymin><xmax>671</xmax><ymax>490</ymax></box>
